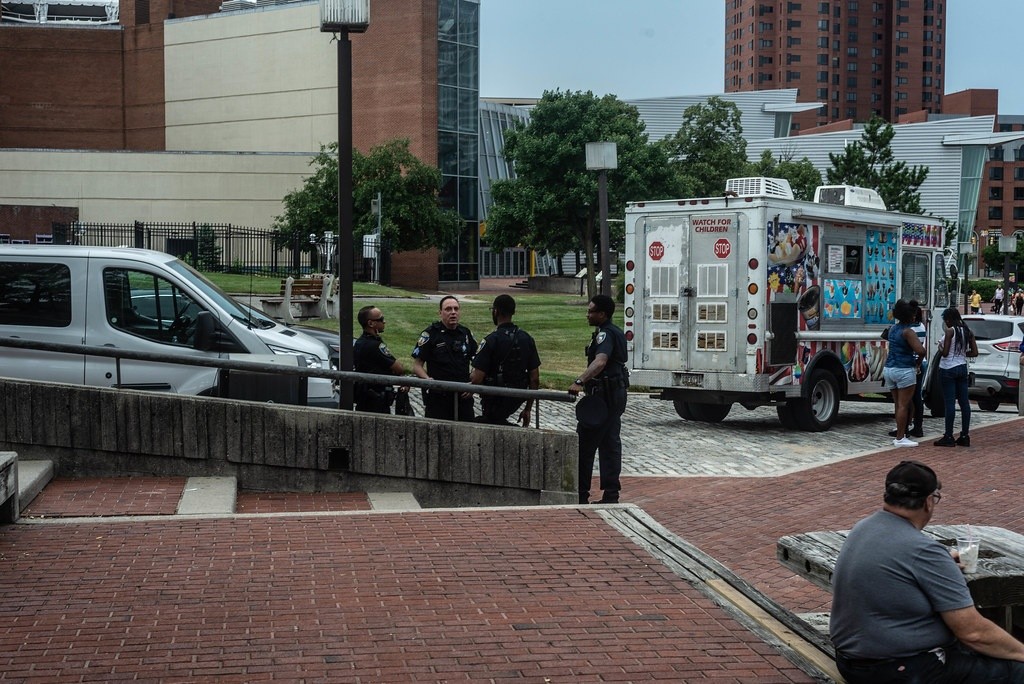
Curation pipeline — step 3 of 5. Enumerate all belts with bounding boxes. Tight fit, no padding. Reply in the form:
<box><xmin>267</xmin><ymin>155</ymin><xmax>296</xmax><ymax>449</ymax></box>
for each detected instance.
<box><xmin>837</xmin><ymin>650</ymin><xmax>895</xmax><ymax>667</ymax></box>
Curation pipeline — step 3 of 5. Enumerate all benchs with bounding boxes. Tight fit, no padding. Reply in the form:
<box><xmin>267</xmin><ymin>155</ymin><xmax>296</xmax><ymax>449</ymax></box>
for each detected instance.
<box><xmin>259</xmin><ymin>277</ymin><xmax>341</xmax><ymax>324</ymax></box>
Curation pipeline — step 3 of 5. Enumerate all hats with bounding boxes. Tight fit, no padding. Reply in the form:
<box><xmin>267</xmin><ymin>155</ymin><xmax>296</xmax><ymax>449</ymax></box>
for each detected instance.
<box><xmin>576</xmin><ymin>396</ymin><xmax>608</xmax><ymax>429</ymax></box>
<box><xmin>885</xmin><ymin>461</ymin><xmax>942</xmax><ymax>498</ymax></box>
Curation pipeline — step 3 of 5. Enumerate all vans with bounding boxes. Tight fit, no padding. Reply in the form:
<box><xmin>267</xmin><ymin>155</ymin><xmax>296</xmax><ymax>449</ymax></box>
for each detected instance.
<box><xmin>0</xmin><ymin>243</ymin><xmax>340</xmax><ymax>411</ymax></box>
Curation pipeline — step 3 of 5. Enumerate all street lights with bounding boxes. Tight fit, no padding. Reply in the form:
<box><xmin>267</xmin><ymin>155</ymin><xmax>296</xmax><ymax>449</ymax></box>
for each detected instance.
<box><xmin>959</xmin><ymin>242</ymin><xmax>973</xmax><ymax>314</ymax></box>
<box><xmin>998</xmin><ymin>236</ymin><xmax>1017</xmax><ymax>314</ymax></box>
<box><xmin>586</xmin><ymin>141</ymin><xmax>619</xmax><ymax>299</ymax></box>
<box><xmin>317</xmin><ymin>0</ymin><xmax>370</xmax><ymax>411</ymax></box>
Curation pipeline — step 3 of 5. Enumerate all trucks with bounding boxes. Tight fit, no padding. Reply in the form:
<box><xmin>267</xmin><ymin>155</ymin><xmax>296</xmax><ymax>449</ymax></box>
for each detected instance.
<box><xmin>624</xmin><ymin>178</ymin><xmax>962</xmax><ymax>432</ymax></box>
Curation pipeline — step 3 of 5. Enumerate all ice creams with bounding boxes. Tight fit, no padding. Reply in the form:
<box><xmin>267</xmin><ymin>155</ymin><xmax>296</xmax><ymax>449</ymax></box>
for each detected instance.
<box><xmin>867</xmin><ymin>244</ymin><xmax>896</xmax><ymax>319</ymax></box>
<box><xmin>825</xmin><ymin>301</ymin><xmax>840</xmax><ymax>317</ymax></box>
<box><xmin>794</xmin><ymin>266</ymin><xmax>806</xmax><ymax>298</ymax></box>
<box><xmin>845</xmin><ymin>346</ymin><xmax>871</xmax><ymax>383</ymax></box>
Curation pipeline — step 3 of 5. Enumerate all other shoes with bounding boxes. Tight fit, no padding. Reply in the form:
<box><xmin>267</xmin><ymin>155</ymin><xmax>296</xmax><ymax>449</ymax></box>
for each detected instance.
<box><xmin>591</xmin><ymin>496</ymin><xmax>618</xmax><ymax>503</ymax></box>
<box><xmin>909</xmin><ymin>427</ymin><xmax>924</xmax><ymax>438</ymax></box>
<box><xmin>578</xmin><ymin>492</ymin><xmax>590</xmax><ymax>504</ymax></box>
<box><xmin>954</xmin><ymin>432</ymin><xmax>971</xmax><ymax>447</ymax></box>
<box><xmin>889</xmin><ymin>429</ymin><xmax>908</xmax><ymax>437</ymax></box>
<box><xmin>934</xmin><ymin>434</ymin><xmax>955</xmax><ymax>447</ymax></box>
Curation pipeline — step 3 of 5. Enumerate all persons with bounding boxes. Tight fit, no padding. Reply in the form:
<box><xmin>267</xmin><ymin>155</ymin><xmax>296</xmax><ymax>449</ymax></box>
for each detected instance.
<box><xmin>882</xmin><ymin>299</ymin><xmax>927</xmax><ymax>446</ymax></box>
<box><xmin>970</xmin><ymin>290</ymin><xmax>982</xmax><ymax>314</ymax></box>
<box><xmin>469</xmin><ymin>295</ymin><xmax>541</xmax><ymax>426</ymax></box>
<box><xmin>1019</xmin><ymin>336</ymin><xmax>1024</xmax><ymax>363</ymax></box>
<box><xmin>993</xmin><ymin>284</ymin><xmax>1004</xmax><ymax>314</ymax></box>
<box><xmin>934</xmin><ymin>308</ymin><xmax>978</xmax><ymax>446</ymax></box>
<box><xmin>829</xmin><ymin>460</ymin><xmax>1024</xmax><ymax>684</ymax></box>
<box><xmin>411</xmin><ymin>296</ymin><xmax>478</xmax><ymax>421</ymax></box>
<box><xmin>568</xmin><ymin>295</ymin><xmax>628</xmax><ymax>504</ymax></box>
<box><xmin>353</xmin><ymin>306</ymin><xmax>409</xmax><ymax>414</ymax></box>
<box><xmin>1008</xmin><ymin>287</ymin><xmax>1024</xmax><ymax>315</ymax></box>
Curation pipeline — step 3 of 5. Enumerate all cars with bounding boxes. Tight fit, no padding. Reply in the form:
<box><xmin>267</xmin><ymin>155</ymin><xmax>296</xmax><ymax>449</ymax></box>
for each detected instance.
<box><xmin>126</xmin><ymin>289</ymin><xmax>358</xmax><ymax>371</ymax></box>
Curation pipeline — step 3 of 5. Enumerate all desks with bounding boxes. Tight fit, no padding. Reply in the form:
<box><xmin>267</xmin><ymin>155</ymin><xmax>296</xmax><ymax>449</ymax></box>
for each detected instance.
<box><xmin>777</xmin><ymin>525</ymin><xmax>1024</xmax><ymax>635</ymax></box>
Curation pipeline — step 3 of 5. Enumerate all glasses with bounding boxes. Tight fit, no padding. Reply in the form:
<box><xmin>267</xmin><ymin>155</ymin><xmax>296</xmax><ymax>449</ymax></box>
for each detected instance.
<box><xmin>929</xmin><ymin>492</ymin><xmax>941</xmax><ymax>503</ymax></box>
<box><xmin>368</xmin><ymin>316</ymin><xmax>384</xmax><ymax>322</ymax></box>
<box><xmin>489</xmin><ymin>307</ymin><xmax>497</xmax><ymax>312</ymax></box>
<box><xmin>588</xmin><ymin>309</ymin><xmax>600</xmax><ymax>314</ymax></box>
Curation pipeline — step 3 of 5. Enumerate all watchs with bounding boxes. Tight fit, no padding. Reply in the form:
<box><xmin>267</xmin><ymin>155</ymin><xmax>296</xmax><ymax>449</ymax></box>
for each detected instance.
<box><xmin>575</xmin><ymin>379</ymin><xmax>584</xmax><ymax>386</ymax></box>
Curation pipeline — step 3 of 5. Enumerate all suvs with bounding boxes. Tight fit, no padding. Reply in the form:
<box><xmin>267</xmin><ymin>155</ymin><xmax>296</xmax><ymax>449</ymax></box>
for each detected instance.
<box><xmin>961</xmin><ymin>314</ymin><xmax>1024</xmax><ymax>411</ymax></box>
